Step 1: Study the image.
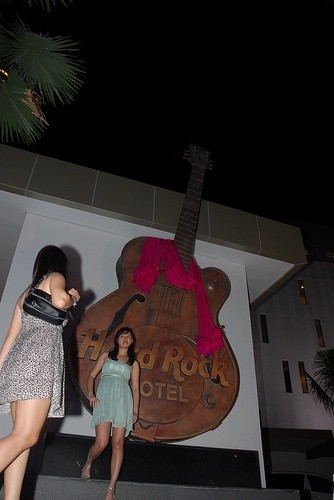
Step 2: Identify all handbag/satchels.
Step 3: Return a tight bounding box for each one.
[23,274,66,325]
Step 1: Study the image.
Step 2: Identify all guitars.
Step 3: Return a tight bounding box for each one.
[63,143,240,442]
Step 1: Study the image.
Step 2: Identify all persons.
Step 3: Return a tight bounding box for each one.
[0,244,81,500]
[81,327,140,499]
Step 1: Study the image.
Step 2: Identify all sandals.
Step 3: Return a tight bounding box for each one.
[81,465,91,480]
[104,488,115,500]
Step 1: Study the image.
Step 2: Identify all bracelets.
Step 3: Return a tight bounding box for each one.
[71,295,77,307]
